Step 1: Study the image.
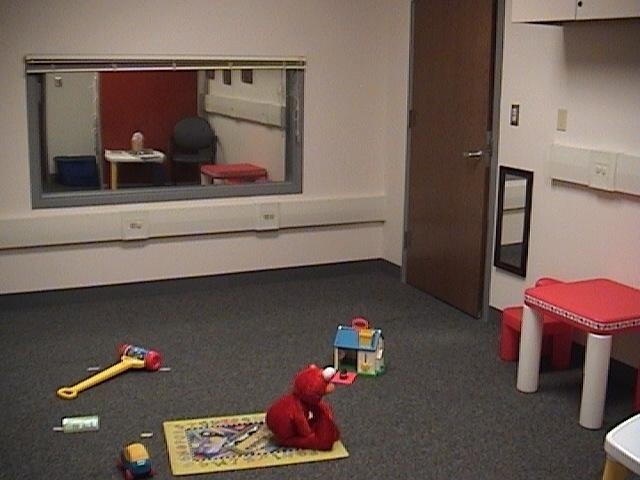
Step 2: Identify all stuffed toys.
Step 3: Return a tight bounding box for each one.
[265,361,341,453]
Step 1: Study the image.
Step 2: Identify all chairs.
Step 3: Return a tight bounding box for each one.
[501,279,572,369]
[166,115,217,186]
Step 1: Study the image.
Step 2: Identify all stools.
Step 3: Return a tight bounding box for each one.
[602,414,640,480]
[105,149,166,190]
[515,278,640,429]
[200,164,268,185]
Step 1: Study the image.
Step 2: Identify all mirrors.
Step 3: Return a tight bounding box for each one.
[493,165,534,278]
[23,54,305,209]
